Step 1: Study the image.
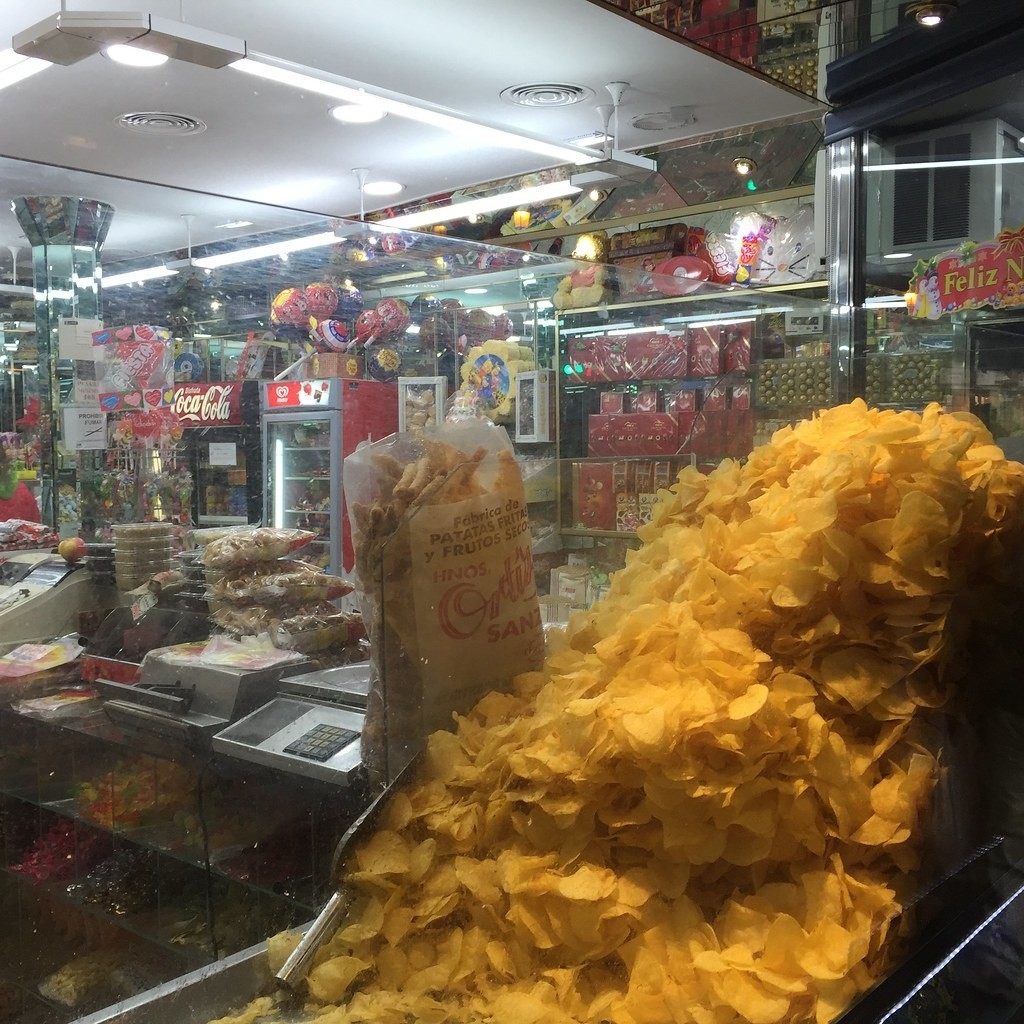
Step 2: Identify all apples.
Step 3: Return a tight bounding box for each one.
[59,537,84,562]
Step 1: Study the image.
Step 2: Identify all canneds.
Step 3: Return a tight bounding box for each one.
[200,448,246,516]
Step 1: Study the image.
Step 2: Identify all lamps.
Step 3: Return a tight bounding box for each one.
[0,0,656,300]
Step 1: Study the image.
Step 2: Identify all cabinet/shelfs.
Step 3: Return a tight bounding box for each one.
[555,280,955,574]
[0,690,374,1024]
[522,457,562,596]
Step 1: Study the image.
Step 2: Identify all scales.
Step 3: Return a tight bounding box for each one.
[211,659,372,787]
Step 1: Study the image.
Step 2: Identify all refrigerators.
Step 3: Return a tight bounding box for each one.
[172,379,262,530]
[259,377,398,612]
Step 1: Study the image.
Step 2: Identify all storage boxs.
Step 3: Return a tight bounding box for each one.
[569,322,752,384]
[589,383,755,457]
[572,462,717,531]
[398,376,448,432]
[515,369,555,443]
[303,353,363,379]
[680,7,762,71]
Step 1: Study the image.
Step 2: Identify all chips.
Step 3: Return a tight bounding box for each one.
[202,398,1024,1024]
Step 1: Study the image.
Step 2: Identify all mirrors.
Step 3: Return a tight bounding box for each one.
[605,0,910,109]
[171,299,555,396]
